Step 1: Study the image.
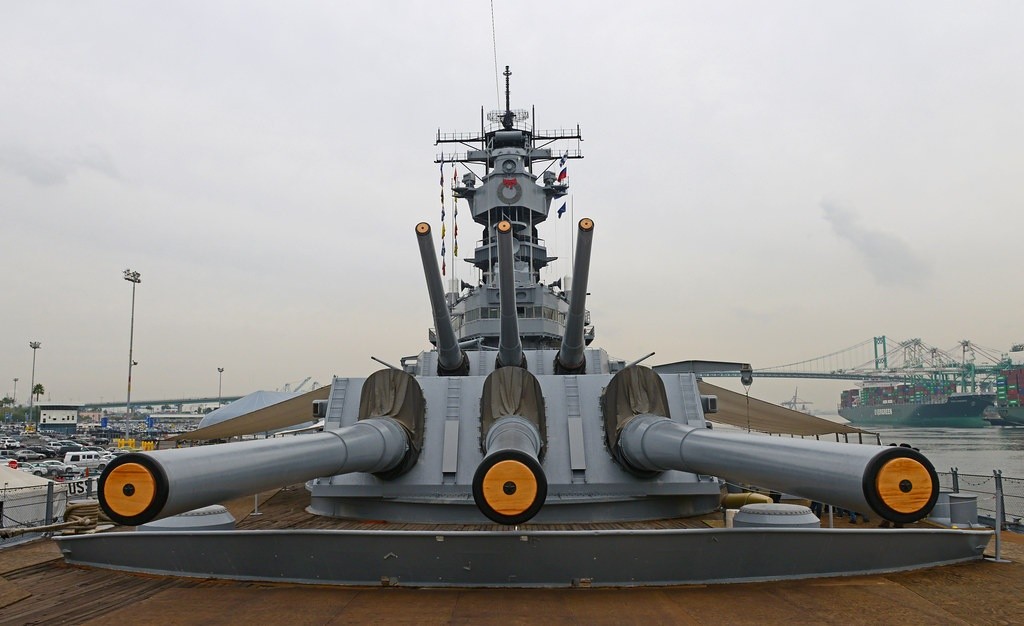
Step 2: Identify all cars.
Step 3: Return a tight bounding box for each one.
[45,460,78,476]
[0,420,111,452]
[15,450,47,460]
[39,461,65,475]
[17,462,48,477]
[100,452,126,462]
[0,450,29,462]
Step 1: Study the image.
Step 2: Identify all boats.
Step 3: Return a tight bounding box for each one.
[777,387,816,416]
[990,359,1024,428]
[836,387,995,428]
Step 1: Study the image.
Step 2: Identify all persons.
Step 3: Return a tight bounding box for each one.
[810,443,920,525]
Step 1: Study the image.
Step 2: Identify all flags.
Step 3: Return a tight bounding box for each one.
[557,203,566,219]
[559,150,568,168]
[558,168,566,182]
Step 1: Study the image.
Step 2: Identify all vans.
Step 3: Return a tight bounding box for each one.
[64,451,108,471]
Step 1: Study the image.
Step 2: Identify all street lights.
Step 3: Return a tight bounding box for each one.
[12,378,19,400]
[217,367,225,407]
[122,267,141,437]
[28,342,42,420]
